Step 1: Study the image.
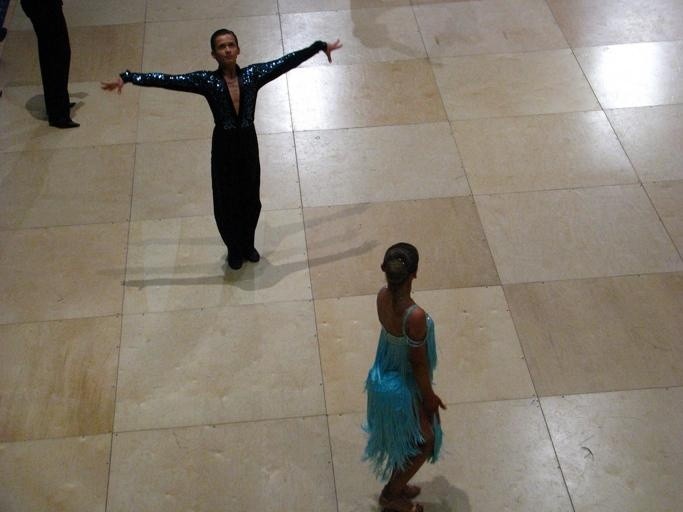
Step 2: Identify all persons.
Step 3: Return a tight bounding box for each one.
[2,0,84,130]
[360,242,448,512]
[99,28,344,271]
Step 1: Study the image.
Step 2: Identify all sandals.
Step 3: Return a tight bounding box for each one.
[379,486,423,512]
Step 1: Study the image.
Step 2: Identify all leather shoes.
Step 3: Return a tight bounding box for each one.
[227,246,260,270]
[47,102,81,128]
